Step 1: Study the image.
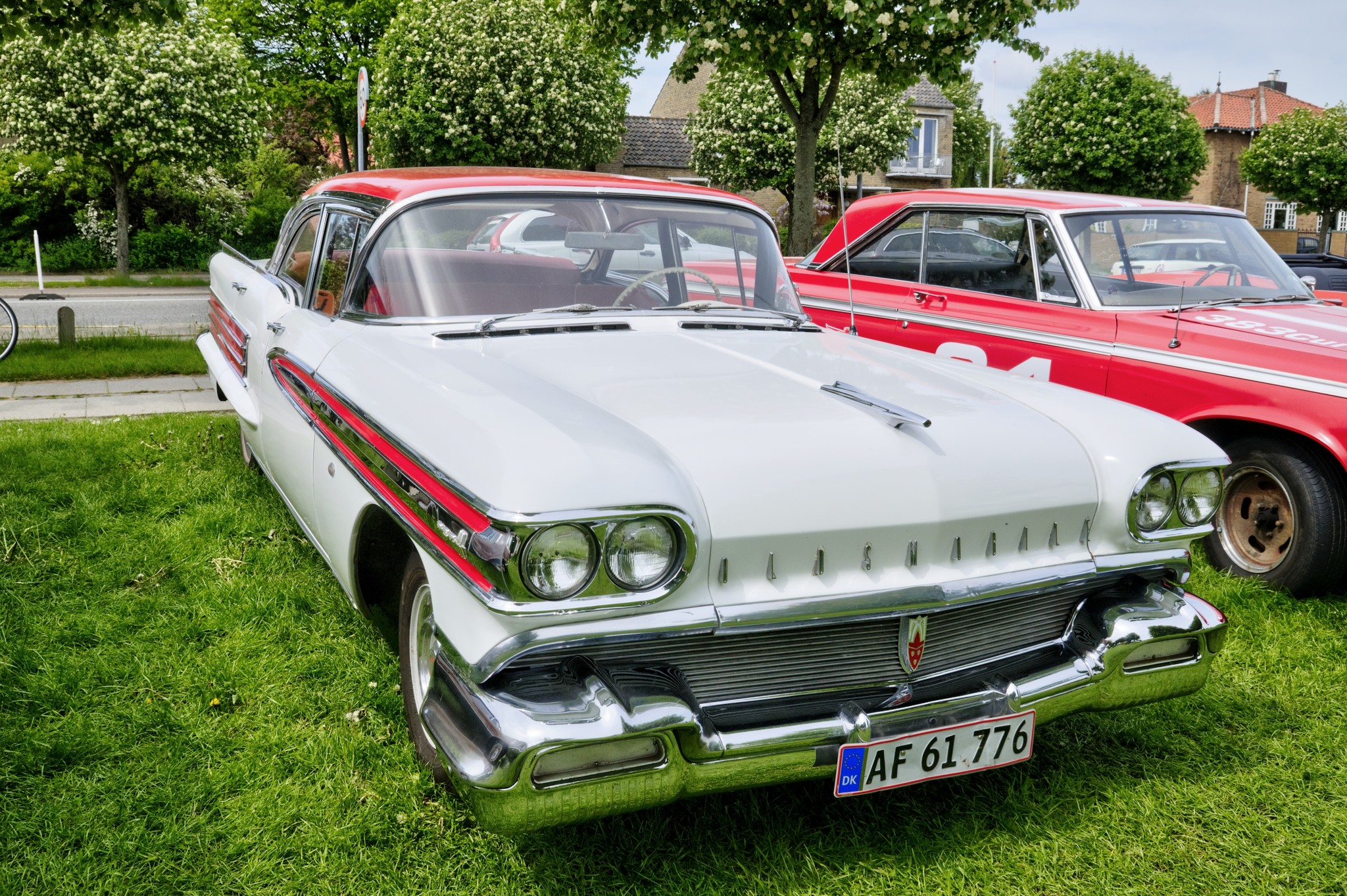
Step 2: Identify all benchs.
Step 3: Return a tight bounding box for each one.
[364,284,669,316]
[850,257,1033,302]
[286,246,578,284]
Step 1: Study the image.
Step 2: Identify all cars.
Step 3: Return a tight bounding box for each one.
[191,162,1232,840]
[658,185,1347,600]
[853,225,1018,260]
[1110,238,1281,288]
[466,209,757,270]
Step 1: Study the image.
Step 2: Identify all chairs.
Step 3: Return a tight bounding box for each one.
[899,159,911,174]
[929,158,945,174]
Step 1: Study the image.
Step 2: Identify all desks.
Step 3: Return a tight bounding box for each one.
[910,156,931,174]
